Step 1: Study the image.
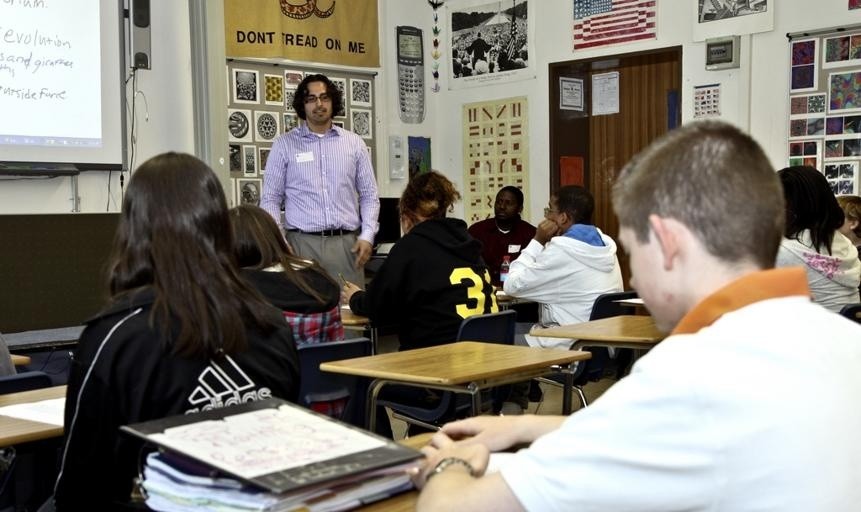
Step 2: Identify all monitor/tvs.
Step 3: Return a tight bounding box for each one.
[373,197,401,256]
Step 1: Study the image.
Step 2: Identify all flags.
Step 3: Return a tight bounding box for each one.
[573,0,655,50]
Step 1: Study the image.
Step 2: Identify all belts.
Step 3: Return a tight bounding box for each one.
[289,229,351,236]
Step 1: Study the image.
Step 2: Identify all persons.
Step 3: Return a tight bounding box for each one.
[0,332,18,379]
[451,23,527,79]
[774,164,861,325]
[341,171,623,442]
[259,74,381,292]
[411,121,861,510]
[31,121,351,510]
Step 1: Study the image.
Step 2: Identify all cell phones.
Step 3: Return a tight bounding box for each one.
[396,25,427,124]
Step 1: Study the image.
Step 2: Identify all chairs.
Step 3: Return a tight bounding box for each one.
[291,334,375,428]
[839,302,861,324]
[525,286,643,416]
[375,309,521,442]
[1,367,55,504]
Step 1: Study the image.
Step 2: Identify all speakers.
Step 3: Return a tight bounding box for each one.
[128,0,151,70]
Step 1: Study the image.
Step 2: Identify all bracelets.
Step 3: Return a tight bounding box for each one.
[425,457,473,481]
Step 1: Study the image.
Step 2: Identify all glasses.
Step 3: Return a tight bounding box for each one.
[544,207,571,221]
[304,94,330,103]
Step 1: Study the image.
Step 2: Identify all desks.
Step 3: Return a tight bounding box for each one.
[530,313,666,416]
[330,424,472,512]
[319,336,592,433]
[0,383,67,450]
[336,305,370,338]
[489,284,538,313]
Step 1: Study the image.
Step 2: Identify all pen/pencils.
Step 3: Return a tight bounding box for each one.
[339,272,349,288]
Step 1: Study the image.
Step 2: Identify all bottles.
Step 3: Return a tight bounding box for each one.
[499,254,511,289]
